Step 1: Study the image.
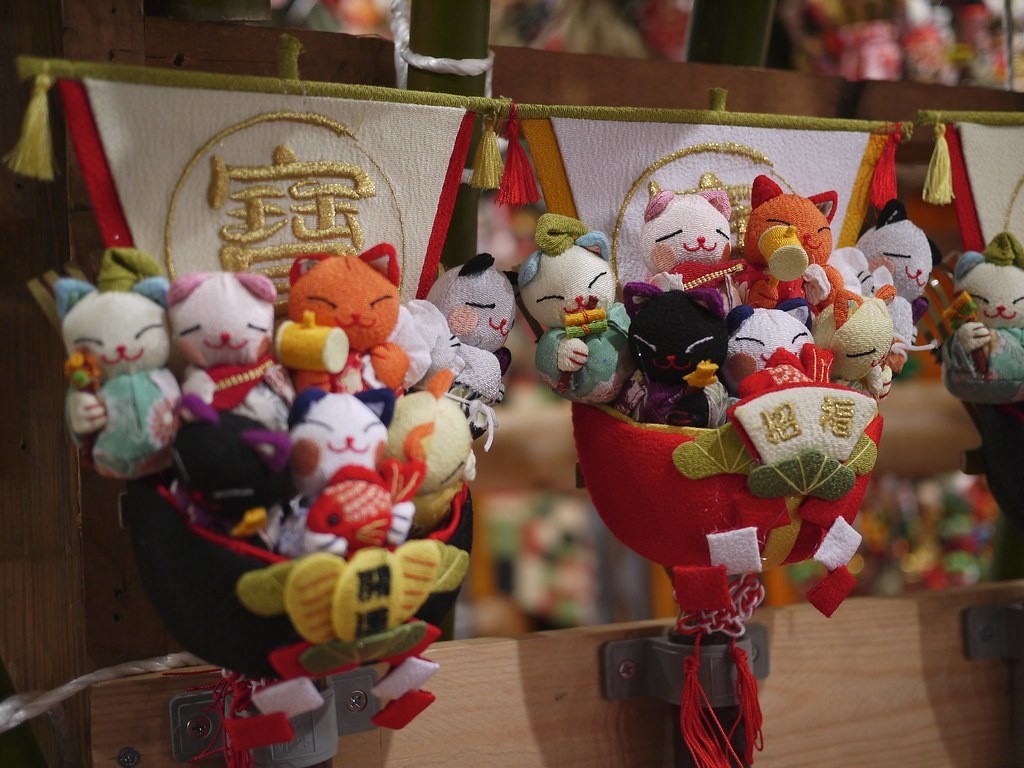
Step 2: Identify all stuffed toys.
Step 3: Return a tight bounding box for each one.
[518,175,943,430]
[55,243,519,564]
[940,231,1024,404]
